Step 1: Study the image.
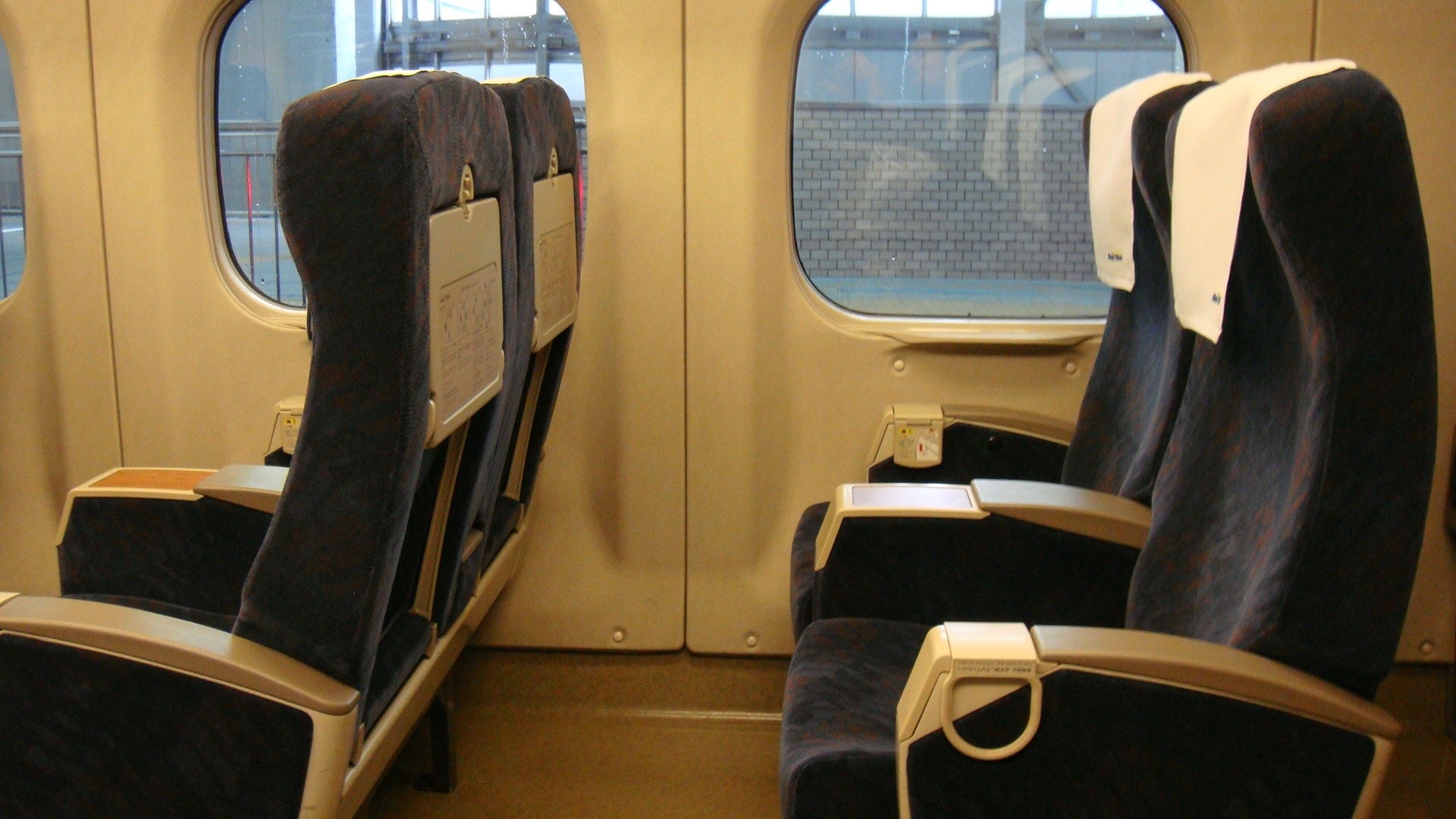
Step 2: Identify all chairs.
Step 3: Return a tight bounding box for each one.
[778,61,1435,819]
[0,69,589,819]
